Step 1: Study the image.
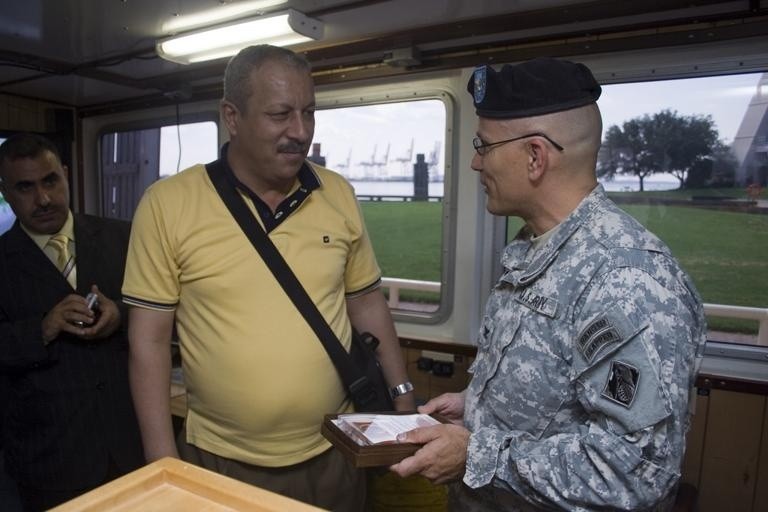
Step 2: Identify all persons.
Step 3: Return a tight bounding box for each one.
[0,135,143,511]
[388,60,706,511]
[121,43,417,512]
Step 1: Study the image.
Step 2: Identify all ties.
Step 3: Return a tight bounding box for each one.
[46,234,76,291]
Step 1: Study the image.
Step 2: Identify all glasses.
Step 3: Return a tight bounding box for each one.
[471,132,564,156]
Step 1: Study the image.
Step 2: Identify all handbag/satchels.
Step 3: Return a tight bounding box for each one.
[349,326,395,414]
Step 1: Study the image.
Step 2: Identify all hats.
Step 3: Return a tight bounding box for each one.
[466,56,602,118]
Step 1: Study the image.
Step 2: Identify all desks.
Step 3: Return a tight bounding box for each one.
[42,456,331,512]
[167,365,187,436]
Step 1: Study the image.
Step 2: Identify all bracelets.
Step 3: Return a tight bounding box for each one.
[388,382,415,400]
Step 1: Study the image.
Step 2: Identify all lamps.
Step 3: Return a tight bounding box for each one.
[153,8,325,66]
